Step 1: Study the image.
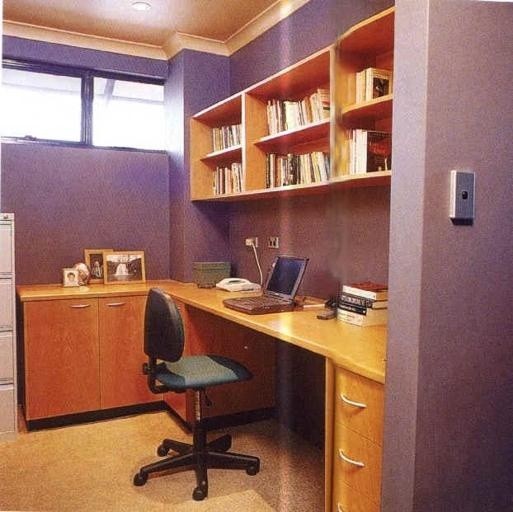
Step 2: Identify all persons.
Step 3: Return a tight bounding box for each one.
[90,260,102,279]
[64,271,77,285]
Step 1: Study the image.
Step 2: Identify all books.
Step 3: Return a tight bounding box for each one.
[209,67,391,195]
[335,279,388,327]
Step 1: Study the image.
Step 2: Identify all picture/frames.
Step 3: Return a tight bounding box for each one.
[82,247,146,286]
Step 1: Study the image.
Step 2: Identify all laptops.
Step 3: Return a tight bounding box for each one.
[223,254,309,315]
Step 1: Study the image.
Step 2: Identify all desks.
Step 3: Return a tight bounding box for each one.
[157,281,387,386]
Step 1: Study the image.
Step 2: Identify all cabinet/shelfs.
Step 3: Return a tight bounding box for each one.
[323,358,384,512]
[161,297,276,424]
[187,6,396,203]
[15,279,183,421]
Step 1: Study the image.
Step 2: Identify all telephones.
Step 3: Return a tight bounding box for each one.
[216,278,262,292]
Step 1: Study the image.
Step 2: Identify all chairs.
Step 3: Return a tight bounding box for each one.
[132,285,262,501]
